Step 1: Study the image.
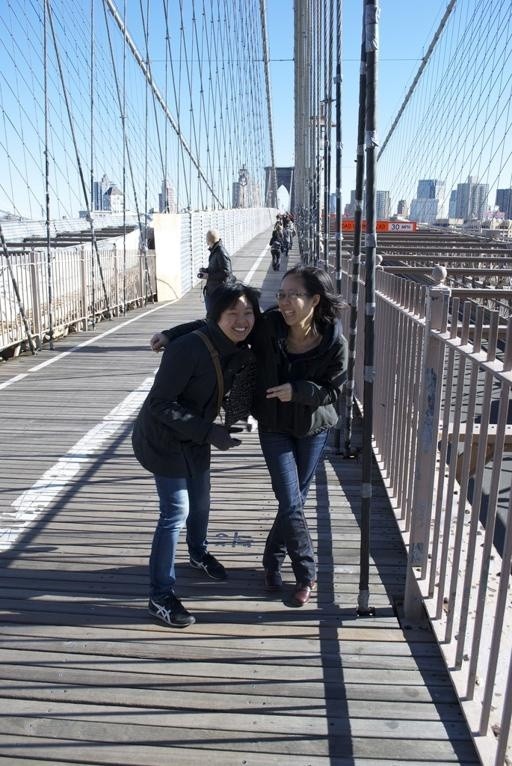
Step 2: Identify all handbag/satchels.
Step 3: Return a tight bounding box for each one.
[225,351,257,426]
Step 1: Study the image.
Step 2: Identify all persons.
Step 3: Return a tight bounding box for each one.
[197,229,233,314]
[269,211,297,272]
[150,266,350,606]
[130,276,261,627]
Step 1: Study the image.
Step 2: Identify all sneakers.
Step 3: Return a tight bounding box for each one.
[189,553,226,580]
[291,579,315,605]
[149,593,196,628]
[263,560,284,591]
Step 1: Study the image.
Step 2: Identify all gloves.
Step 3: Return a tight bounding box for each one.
[207,423,242,450]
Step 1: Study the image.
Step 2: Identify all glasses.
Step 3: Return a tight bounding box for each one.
[275,290,311,300]
